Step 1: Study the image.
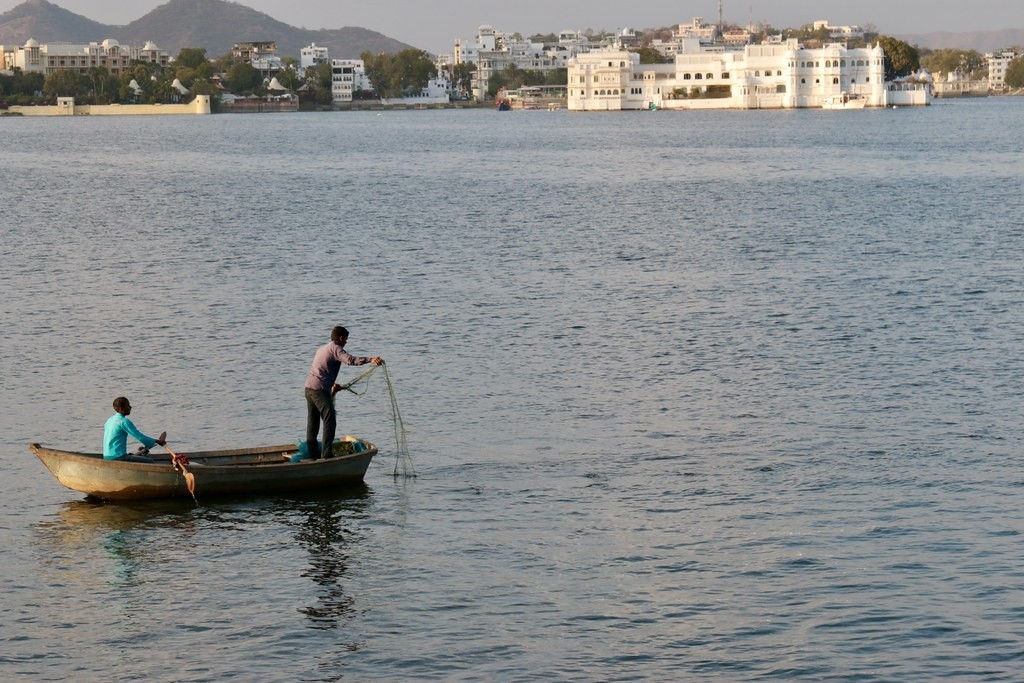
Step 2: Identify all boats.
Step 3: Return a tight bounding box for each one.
[28,436,380,505]
[820,92,869,110]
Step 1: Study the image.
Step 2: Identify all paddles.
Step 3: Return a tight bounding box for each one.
[159,431,195,494]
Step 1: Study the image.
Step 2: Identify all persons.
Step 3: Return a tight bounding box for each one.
[305,326,385,460]
[103,396,167,464]
